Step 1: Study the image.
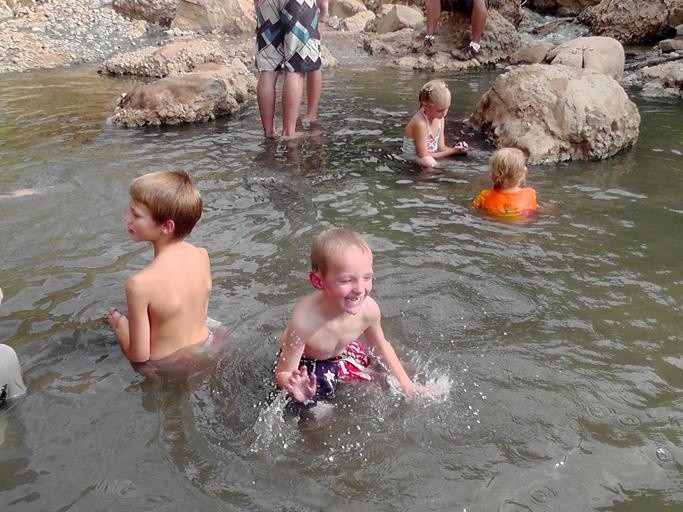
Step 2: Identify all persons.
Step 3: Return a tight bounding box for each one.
[256,0,322,159]
[272,228,438,436]
[0,288,27,447]
[297,0,327,144]
[402,80,469,171]
[104,168,228,383]
[423,0,492,60]
[470,147,540,228]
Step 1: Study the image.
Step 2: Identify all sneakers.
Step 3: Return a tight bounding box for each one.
[423,38,437,54]
[451,45,481,60]
